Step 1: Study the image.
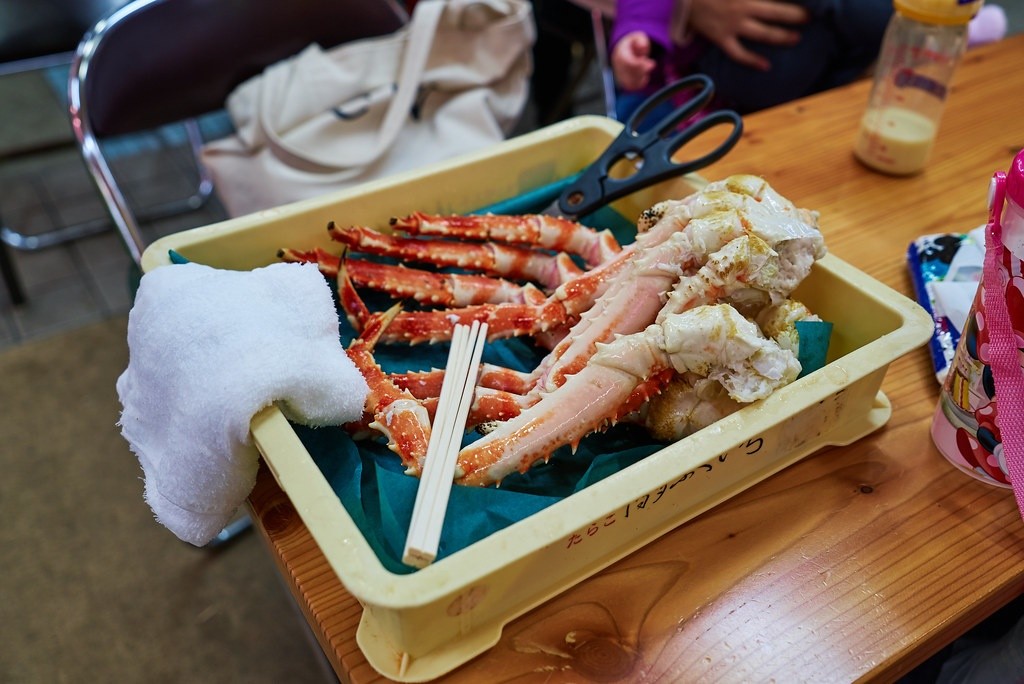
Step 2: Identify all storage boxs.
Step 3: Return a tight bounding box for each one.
[138,114,934,683]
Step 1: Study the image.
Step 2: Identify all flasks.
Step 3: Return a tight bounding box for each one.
[923,151,1024,496]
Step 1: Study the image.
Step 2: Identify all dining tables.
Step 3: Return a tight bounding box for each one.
[242,28,1024,682]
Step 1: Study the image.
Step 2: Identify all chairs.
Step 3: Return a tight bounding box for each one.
[65,0,410,261]
[1,1,211,305]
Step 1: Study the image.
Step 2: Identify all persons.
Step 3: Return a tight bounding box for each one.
[532,1,890,144]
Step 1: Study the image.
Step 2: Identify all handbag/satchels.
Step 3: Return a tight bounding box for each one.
[199,0,536,221]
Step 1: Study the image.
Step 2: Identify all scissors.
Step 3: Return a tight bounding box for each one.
[537,73,744,223]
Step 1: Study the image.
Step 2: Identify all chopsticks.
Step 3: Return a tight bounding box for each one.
[402,322,490,568]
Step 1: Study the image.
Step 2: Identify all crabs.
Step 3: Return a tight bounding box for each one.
[275,175,829,488]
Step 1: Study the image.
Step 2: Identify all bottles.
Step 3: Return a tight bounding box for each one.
[853,0,980,179]
